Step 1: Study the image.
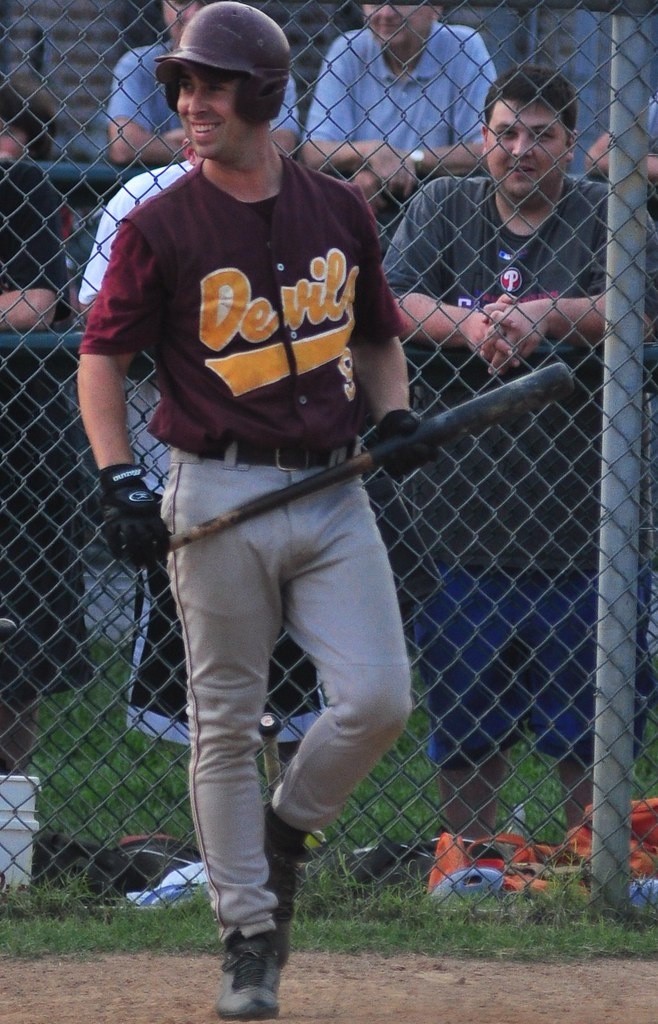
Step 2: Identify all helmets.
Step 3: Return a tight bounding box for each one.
[154,1,291,123]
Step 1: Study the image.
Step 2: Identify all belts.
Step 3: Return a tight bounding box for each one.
[198,434,357,471]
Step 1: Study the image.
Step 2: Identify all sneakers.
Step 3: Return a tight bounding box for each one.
[216,931,282,1019]
[263,800,308,970]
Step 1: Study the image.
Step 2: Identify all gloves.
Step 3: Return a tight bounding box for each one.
[98,463,172,574]
[378,409,442,466]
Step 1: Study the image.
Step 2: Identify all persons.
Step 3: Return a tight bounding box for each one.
[0,81,92,776]
[106,0,301,495]
[300,1,497,215]
[76,0,437,1024]
[380,65,658,842]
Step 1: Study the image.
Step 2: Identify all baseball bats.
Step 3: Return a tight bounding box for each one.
[165,357,579,554]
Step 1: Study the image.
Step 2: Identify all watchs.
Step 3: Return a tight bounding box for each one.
[409,150,425,171]
